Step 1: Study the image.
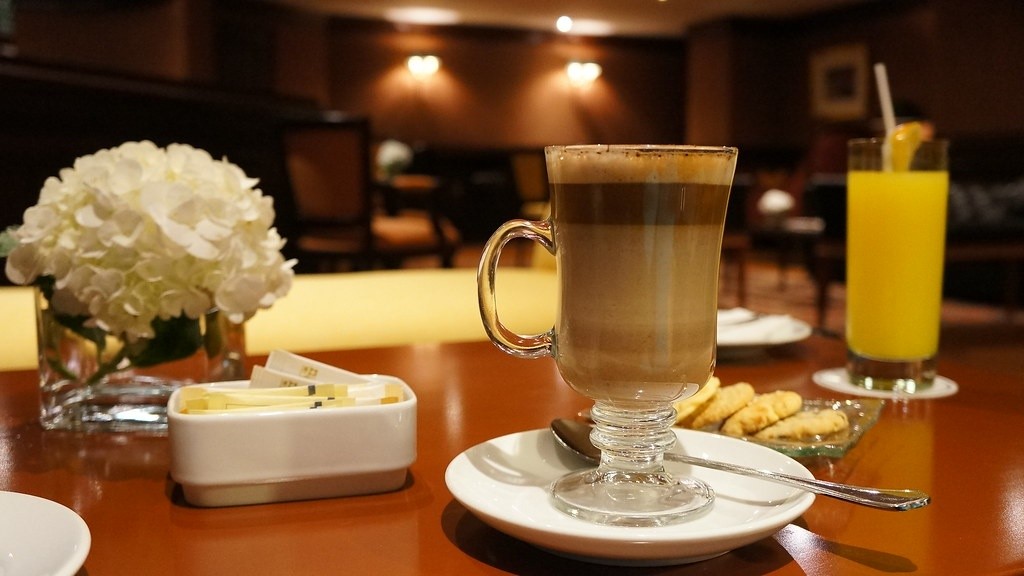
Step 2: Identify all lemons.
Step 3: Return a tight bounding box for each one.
[890,120,923,173]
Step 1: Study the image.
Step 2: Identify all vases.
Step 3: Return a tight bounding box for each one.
[34,276,244,436]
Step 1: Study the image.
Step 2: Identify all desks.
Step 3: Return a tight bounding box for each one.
[0,328,1024,576]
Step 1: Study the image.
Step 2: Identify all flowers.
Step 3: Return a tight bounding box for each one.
[5,141,295,334]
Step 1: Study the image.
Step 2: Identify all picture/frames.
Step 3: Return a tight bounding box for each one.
[809,39,875,122]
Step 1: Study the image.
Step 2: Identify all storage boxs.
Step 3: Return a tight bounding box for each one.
[167,373,419,508]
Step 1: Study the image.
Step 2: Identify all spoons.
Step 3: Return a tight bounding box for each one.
[551,420,932,512]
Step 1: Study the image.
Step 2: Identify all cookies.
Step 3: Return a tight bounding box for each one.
[668,377,850,438]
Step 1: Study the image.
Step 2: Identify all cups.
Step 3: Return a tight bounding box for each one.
[476,144,738,527]
[845,137,949,390]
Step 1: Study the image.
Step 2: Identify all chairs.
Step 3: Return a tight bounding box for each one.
[718,184,847,325]
[279,112,463,269]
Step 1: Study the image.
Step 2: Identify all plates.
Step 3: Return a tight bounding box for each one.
[714,398,886,460]
[717,308,813,359]
[0,492,92,576]
[445,426,817,565]
